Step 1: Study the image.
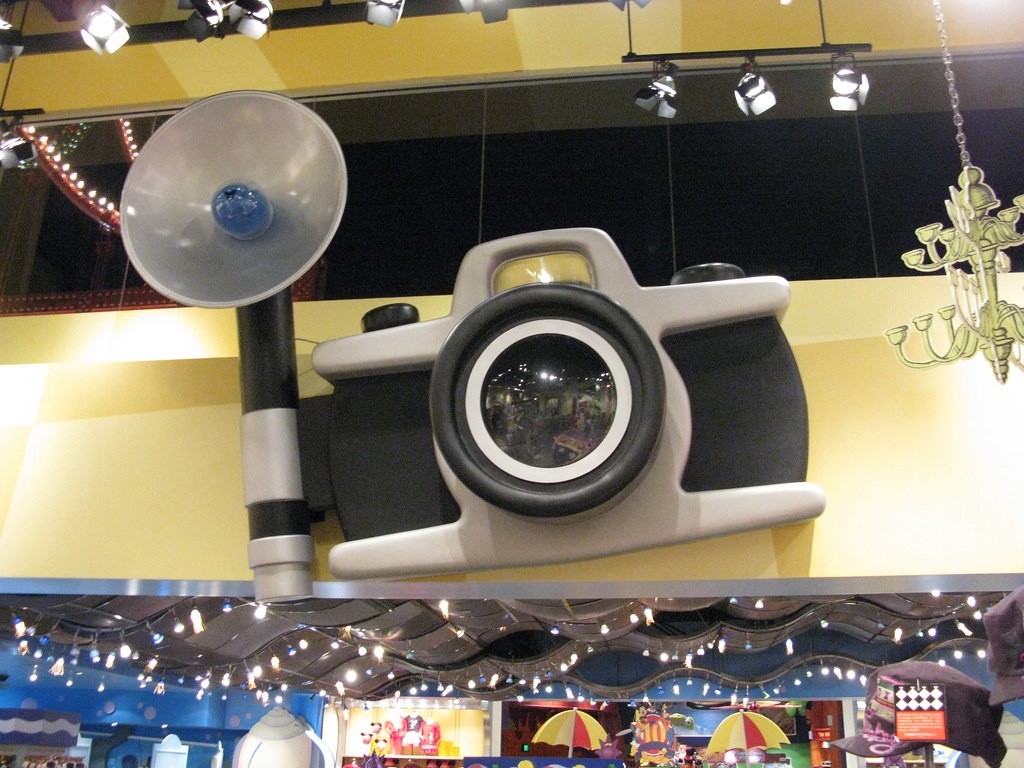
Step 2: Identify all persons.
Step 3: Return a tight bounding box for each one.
[361,711,442,754]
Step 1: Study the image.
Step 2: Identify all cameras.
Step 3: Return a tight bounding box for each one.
[309,226,833,580]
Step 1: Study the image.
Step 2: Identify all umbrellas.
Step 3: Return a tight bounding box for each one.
[706,709,791,768]
[531,707,610,759]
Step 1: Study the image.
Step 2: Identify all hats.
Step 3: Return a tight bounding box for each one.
[828,660,1007,768]
[983,586,1024,706]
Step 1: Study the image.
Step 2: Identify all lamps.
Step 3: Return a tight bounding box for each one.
[456,0,510,25]
[885,165,1024,384]
[178,0,276,44]
[633,63,680,120]
[733,56,776,118]
[359,0,406,28]
[609,0,652,11]
[826,50,871,113]
[44,0,135,54]
[0,11,27,67]
[0,114,43,172]
